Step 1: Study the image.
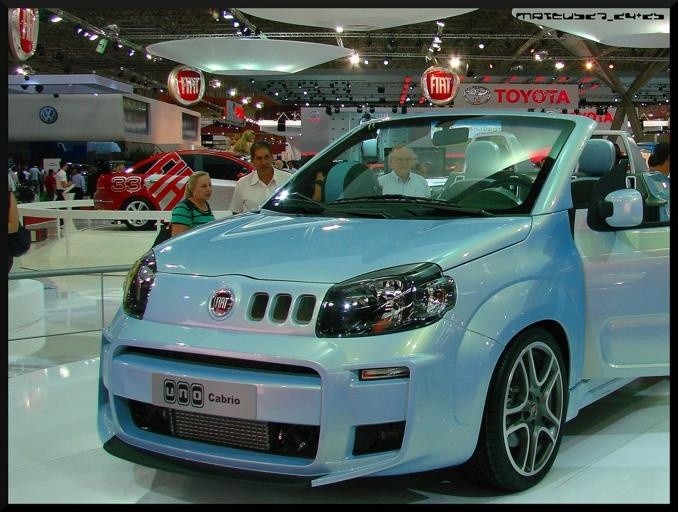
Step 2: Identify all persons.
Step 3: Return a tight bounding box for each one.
[377,145,431,199]
[229,129,257,156]
[647,154,661,171]
[650,142,671,179]
[8,162,124,212]
[287,161,298,175]
[171,171,216,237]
[229,141,291,214]
[274,159,284,170]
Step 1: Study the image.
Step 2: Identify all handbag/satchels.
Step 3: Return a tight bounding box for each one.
[151,201,193,249]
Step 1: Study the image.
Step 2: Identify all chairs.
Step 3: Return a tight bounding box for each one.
[572,138,631,209]
[465,142,504,181]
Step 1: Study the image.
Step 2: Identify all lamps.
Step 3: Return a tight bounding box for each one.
[44,8,566,110]
